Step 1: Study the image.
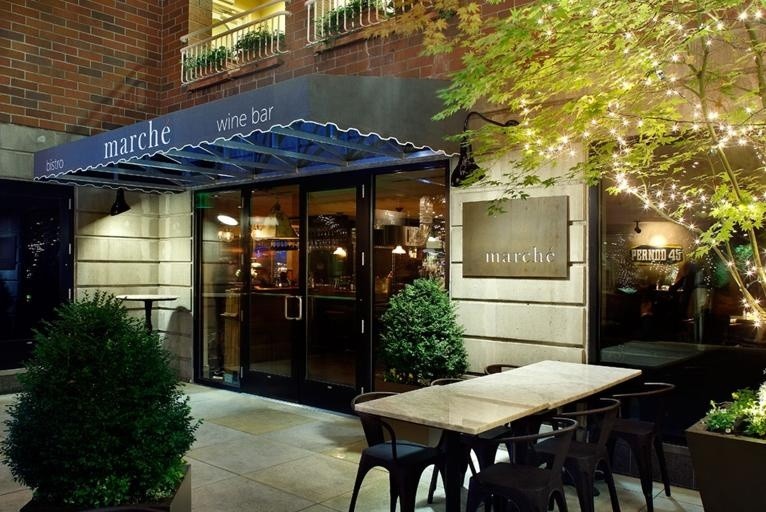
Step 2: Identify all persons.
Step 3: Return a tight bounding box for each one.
[281,268,296,286]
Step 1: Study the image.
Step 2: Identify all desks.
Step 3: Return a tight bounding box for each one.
[114,291,177,337]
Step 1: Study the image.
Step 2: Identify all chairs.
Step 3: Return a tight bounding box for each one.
[350,363,675,511]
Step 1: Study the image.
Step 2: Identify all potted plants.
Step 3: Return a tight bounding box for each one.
[312,1,426,38]
[684,379,765,512]
[373,272,470,446]
[181,29,286,82]
[0,287,204,512]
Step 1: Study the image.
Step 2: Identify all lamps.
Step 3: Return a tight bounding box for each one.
[451,110,521,189]
